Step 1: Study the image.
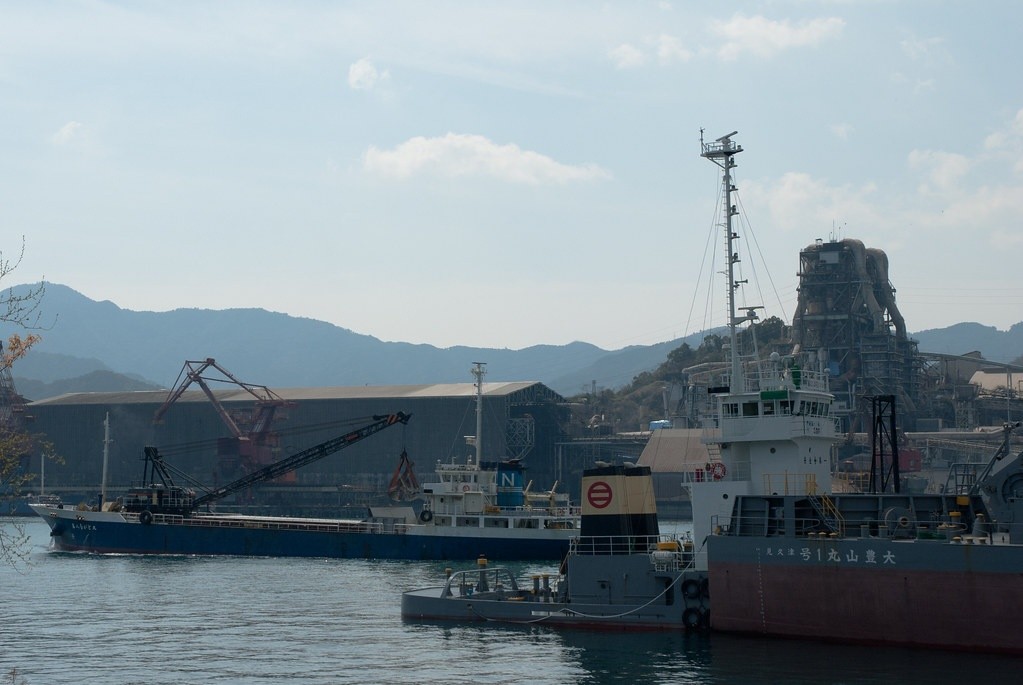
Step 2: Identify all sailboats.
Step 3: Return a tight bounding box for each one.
[27,361,585,561]
[682,131,844,569]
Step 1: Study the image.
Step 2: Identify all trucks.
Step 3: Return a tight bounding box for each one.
[649,420,672,432]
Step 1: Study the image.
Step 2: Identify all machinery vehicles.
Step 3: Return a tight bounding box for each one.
[120,412,413,516]
[153,358,287,465]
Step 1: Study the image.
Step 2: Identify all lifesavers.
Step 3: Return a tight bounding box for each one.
[139,510,153,526]
[681,579,711,631]
[711,462,727,479]
[463,485,470,492]
[420,509,433,522]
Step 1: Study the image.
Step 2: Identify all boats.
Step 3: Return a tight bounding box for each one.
[706,394,1021,654]
[396,465,709,641]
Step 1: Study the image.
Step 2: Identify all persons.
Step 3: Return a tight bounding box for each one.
[57,502,63,509]
